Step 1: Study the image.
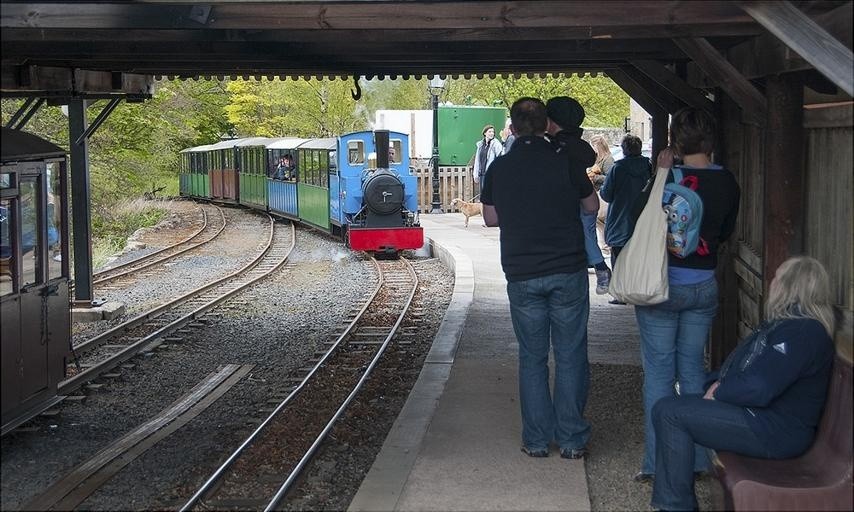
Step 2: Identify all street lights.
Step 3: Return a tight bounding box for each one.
[426,76,447,214]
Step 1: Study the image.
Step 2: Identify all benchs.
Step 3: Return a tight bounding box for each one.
[710,355,854,512]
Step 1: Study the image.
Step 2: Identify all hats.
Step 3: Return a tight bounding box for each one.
[545,96,585,129]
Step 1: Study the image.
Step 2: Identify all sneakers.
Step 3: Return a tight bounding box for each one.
[596,269,611,295]
[520,441,548,457]
[561,448,585,459]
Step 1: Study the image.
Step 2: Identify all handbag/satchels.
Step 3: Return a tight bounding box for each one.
[607,209,670,307]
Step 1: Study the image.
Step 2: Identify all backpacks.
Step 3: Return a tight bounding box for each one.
[666,169,710,259]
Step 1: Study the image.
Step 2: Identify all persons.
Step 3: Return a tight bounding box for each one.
[650,254,834,511]
[586,134,616,273]
[473,125,503,183]
[600,133,652,306]
[502,118,516,153]
[545,96,612,295]
[278,154,297,180]
[388,147,396,163]
[626,107,741,485]
[479,96,599,459]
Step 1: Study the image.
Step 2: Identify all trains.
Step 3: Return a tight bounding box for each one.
[177,127,424,259]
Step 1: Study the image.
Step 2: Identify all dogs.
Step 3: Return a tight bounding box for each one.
[449,197,483,229]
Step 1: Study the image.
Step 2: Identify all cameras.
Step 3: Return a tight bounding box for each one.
[673,153,683,164]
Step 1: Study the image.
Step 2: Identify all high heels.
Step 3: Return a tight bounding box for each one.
[636,474,655,487]
